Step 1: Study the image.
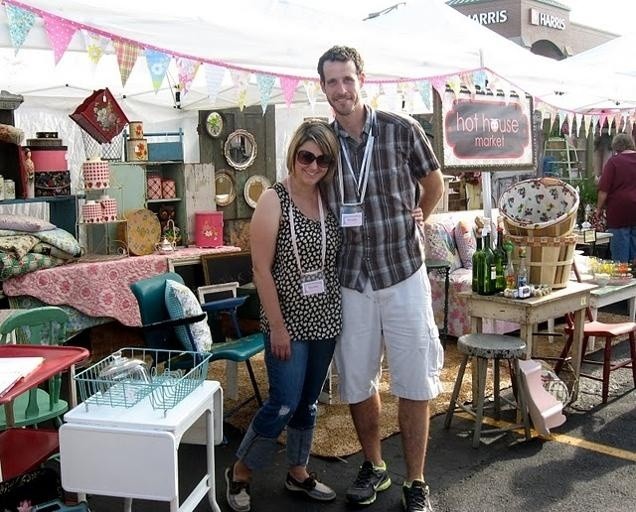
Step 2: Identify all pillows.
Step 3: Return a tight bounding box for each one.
[424,214,463,273]
[0,217,57,232]
[453,217,476,269]
[164,276,214,353]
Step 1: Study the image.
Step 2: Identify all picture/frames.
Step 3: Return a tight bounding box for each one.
[200,249,261,340]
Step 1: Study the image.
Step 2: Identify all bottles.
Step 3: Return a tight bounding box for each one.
[0,175,15,201]
[517,246,529,288]
[505,251,515,288]
[471,229,507,296]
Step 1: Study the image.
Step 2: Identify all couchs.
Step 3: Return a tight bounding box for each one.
[420,208,520,340]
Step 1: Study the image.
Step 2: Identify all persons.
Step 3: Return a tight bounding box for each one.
[591,131,636,268]
[224,118,426,510]
[312,48,447,511]
[22,147,39,201]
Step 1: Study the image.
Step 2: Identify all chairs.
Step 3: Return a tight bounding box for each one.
[551,258,636,403]
[133,272,272,427]
[0,306,69,435]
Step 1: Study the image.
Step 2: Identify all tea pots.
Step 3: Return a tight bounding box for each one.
[153,236,176,254]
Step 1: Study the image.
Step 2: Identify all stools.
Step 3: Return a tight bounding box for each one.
[442,332,533,449]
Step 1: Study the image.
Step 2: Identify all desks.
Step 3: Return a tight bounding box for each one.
[574,272,636,350]
[463,283,596,443]
[3,243,242,342]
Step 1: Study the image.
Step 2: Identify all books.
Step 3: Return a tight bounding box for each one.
[0,355,45,399]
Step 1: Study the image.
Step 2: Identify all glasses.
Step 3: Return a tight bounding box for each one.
[296,150,331,168]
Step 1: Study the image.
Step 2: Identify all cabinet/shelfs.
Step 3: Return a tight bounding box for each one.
[0,342,85,512]
[71,161,129,262]
[0,89,50,221]
[424,256,451,347]
[58,377,226,512]
[107,162,188,248]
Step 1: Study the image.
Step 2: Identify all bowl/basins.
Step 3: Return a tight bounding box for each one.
[596,274,610,288]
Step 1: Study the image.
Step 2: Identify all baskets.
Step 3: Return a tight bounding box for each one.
[71,347,212,417]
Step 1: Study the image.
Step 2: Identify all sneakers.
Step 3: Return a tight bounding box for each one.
[401,478,433,512]
[225,467,252,511]
[346,459,392,507]
[285,472,337,501]
